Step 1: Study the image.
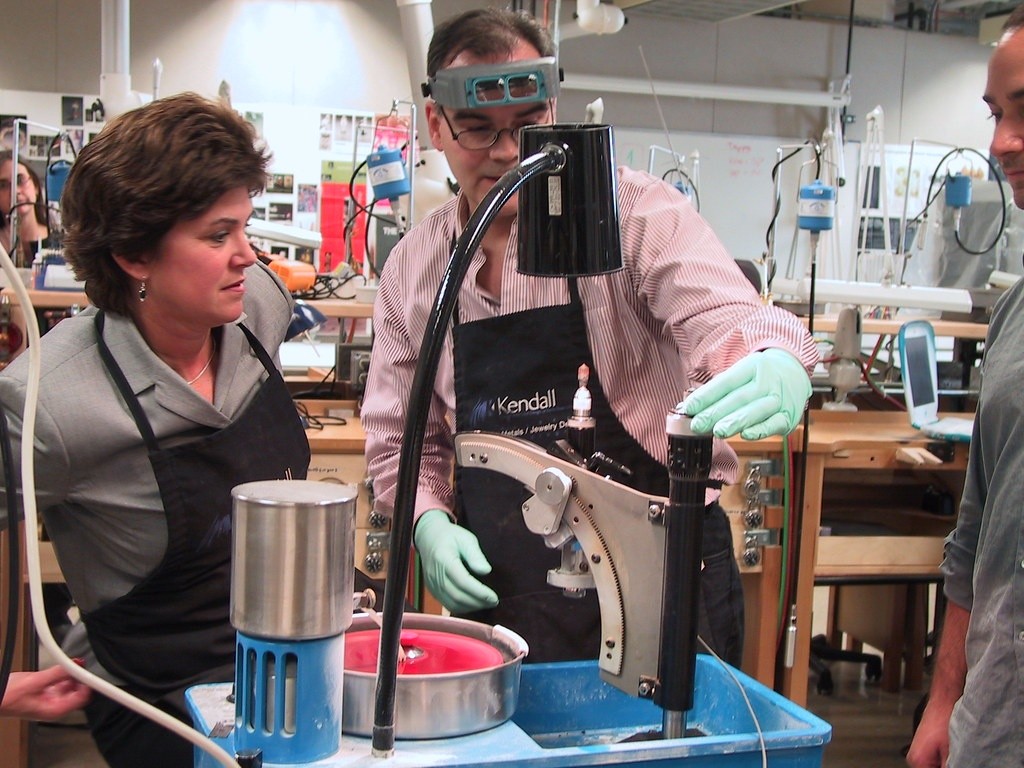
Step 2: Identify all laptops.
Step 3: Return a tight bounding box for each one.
[898,320,975,441]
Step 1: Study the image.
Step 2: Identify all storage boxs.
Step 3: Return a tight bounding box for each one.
[184,654,832,768]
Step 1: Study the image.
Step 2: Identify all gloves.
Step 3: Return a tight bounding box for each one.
[416,509,499,614]
[678,348,813,440]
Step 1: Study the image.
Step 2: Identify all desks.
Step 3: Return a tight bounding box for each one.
[0,287,989,768]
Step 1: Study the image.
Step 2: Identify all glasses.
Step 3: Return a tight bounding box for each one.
[440,101,555,150]
[0,174,33,190]
[465,69,549,107]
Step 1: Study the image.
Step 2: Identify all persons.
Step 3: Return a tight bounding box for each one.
[907,2,1024,768]
[0,153,66,269]
[359,7,818,665]
[0,92,311,768]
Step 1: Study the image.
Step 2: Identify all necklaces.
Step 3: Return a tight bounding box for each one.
[187,337,217,385]
